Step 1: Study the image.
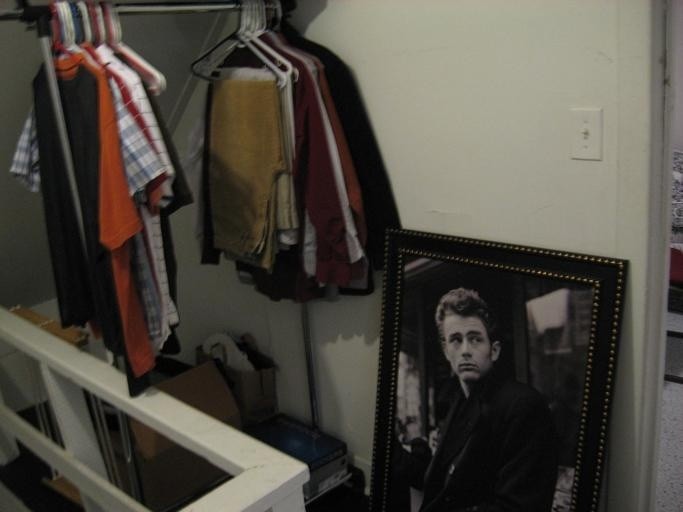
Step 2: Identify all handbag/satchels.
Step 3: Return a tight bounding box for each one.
[194,329,280,428]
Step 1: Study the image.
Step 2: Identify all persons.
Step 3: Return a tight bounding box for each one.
[396,418,429,511]
[425,427,440,456]
[397,288,559,511]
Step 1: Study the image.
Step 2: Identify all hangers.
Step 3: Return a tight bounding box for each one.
[52,1,301,98]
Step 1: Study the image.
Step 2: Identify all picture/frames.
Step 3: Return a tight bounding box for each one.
[366,224,630,512]
